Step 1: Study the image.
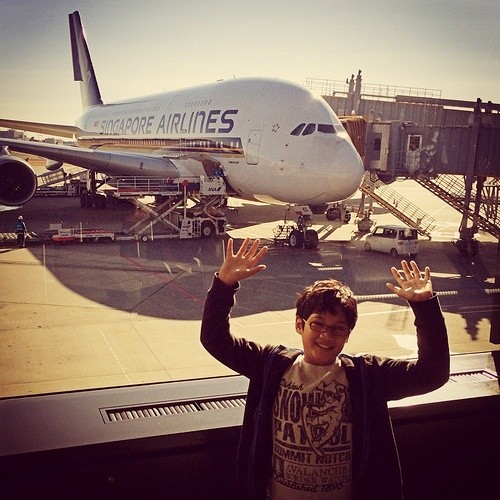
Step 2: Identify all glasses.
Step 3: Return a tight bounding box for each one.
[302,319,351,336]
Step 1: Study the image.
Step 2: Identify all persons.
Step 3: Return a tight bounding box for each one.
[345,73,355,99]
[200,236,450,500]
[355,69,363,101]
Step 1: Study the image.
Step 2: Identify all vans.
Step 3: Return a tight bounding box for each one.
[364,224,419,259]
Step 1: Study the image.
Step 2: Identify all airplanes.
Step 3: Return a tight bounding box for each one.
[0,11,363,249]
